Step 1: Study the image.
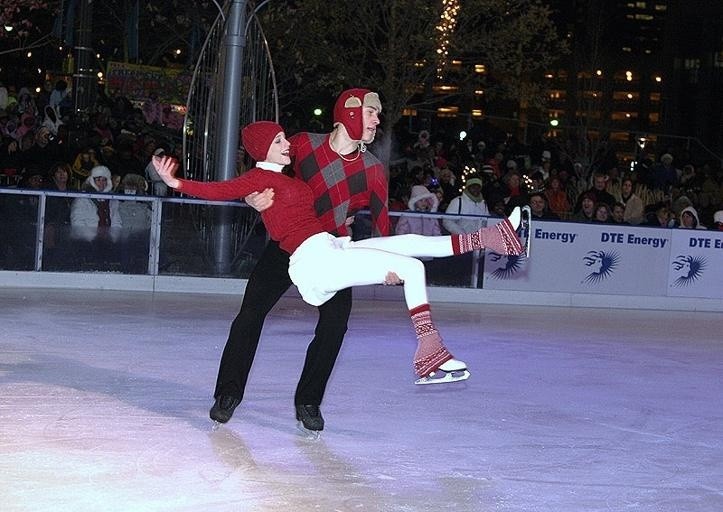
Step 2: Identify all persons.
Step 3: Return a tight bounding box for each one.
[235,144,246,178]
[2,77,184,275]
[388,127,723,231]
[149,120,533,386]
[394,176,490,287]
[206,86,403,433]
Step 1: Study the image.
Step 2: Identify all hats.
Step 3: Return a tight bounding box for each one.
[581,193,596,206]
[333,89,382,144]
[466,176,482,190]
[241,121,284,161]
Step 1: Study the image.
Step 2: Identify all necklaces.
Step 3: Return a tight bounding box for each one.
[330,132,361,162]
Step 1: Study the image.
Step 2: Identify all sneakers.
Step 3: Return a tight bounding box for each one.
[429,358,467,377]
[296,404,324,430]
[210,395,241,423]
[508,206,521,232]
